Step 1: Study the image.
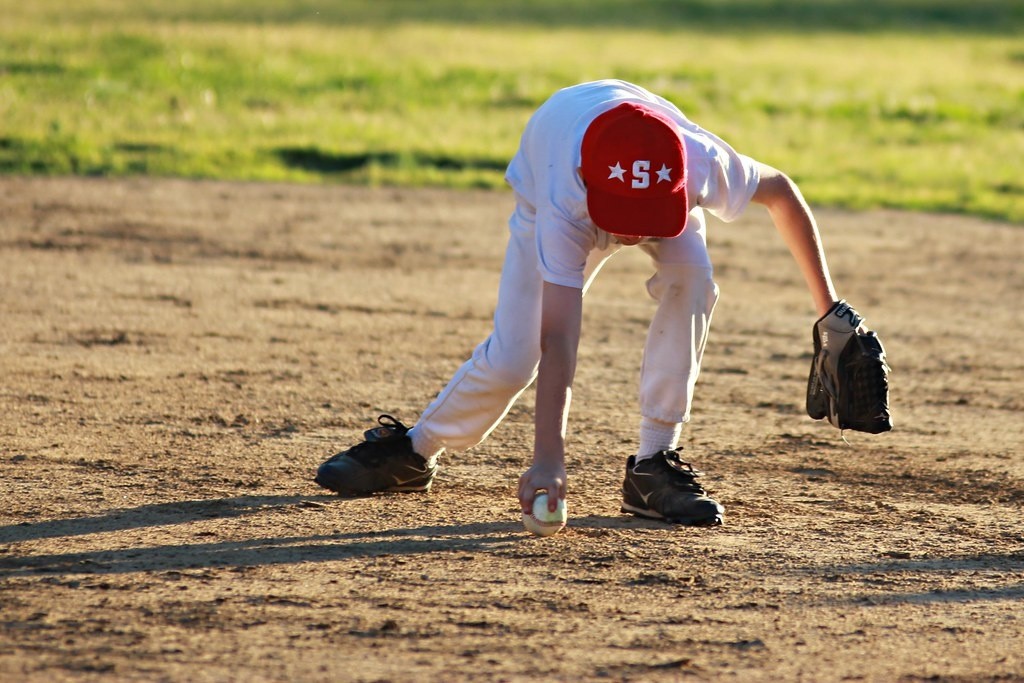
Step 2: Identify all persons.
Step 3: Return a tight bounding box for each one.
[314,79,892,528]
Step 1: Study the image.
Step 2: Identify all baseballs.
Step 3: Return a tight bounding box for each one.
[521,491,568,538]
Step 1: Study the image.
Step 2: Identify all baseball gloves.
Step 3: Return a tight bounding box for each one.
[803,299,895,436]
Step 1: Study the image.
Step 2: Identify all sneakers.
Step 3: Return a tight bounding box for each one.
[314,414,439,492]
[620,446,725,526]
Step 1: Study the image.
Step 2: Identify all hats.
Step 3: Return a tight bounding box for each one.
[579,103,689,239]
